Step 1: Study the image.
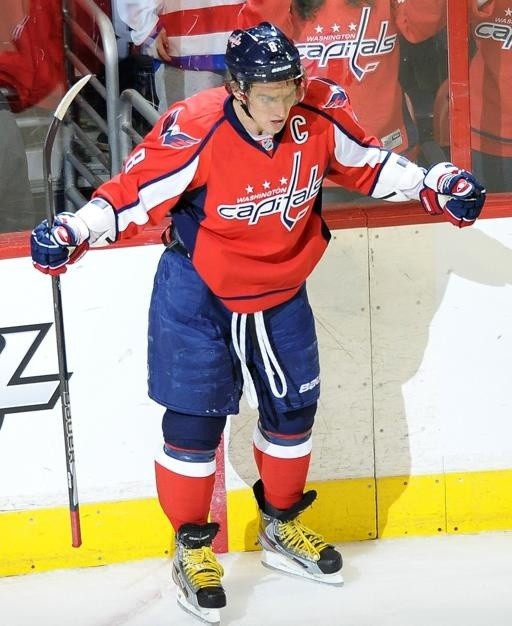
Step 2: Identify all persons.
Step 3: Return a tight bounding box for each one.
[28,19,488,612]
[0,0,511,201]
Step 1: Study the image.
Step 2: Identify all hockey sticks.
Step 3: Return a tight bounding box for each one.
[44,74,94,549]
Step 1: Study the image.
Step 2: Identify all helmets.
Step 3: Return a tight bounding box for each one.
[226,22,308,105]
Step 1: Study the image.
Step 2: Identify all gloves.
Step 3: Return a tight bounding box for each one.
[31,197,119,276]
[419,161,485,225]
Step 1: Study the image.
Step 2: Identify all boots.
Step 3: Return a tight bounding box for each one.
[253,479,342,573]
[174,521,226,610]
